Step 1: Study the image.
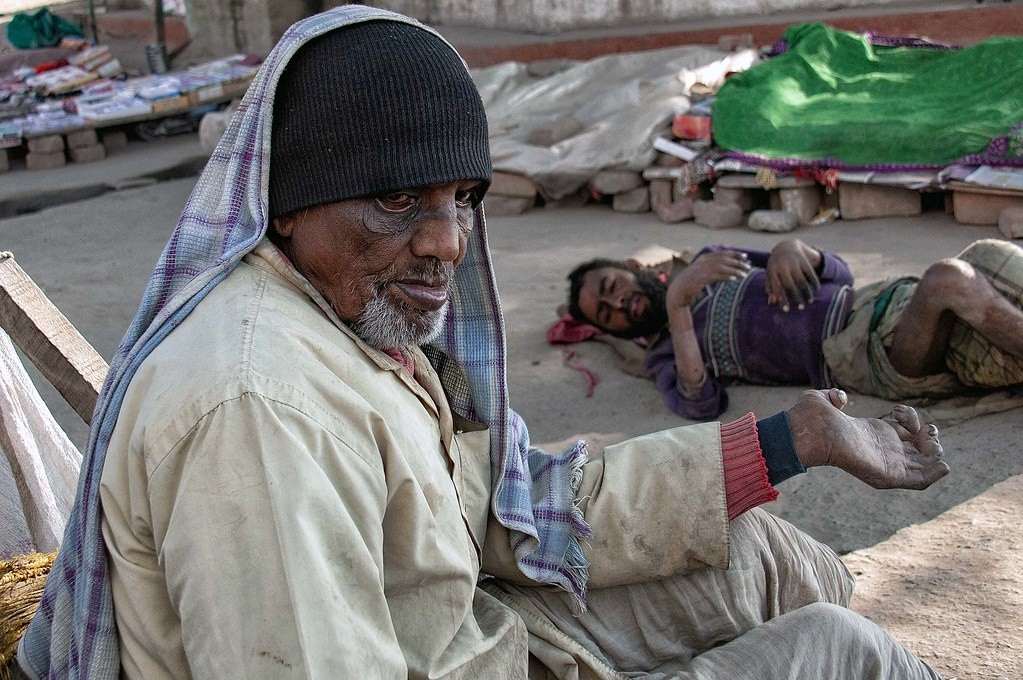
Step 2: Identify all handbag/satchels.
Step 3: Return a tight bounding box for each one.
[68,45,127,81]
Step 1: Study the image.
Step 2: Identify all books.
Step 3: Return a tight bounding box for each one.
[0,44,259,141]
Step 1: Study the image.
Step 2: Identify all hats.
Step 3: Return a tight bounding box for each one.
[267,18,492,211]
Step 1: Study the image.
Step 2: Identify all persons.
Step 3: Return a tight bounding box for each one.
[17,6,951,680]
[566,239,1023,421]
